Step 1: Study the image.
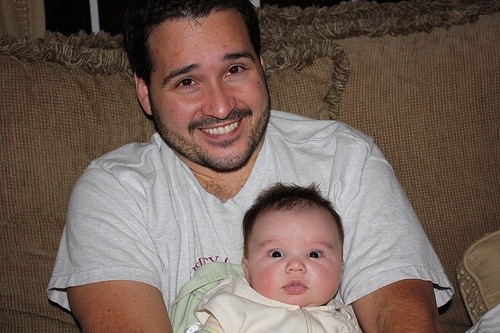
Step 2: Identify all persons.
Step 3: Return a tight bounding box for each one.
[194,181,365,333]
[48,0,455,332]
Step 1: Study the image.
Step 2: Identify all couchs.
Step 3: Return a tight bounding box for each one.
[0,0,500,332]
